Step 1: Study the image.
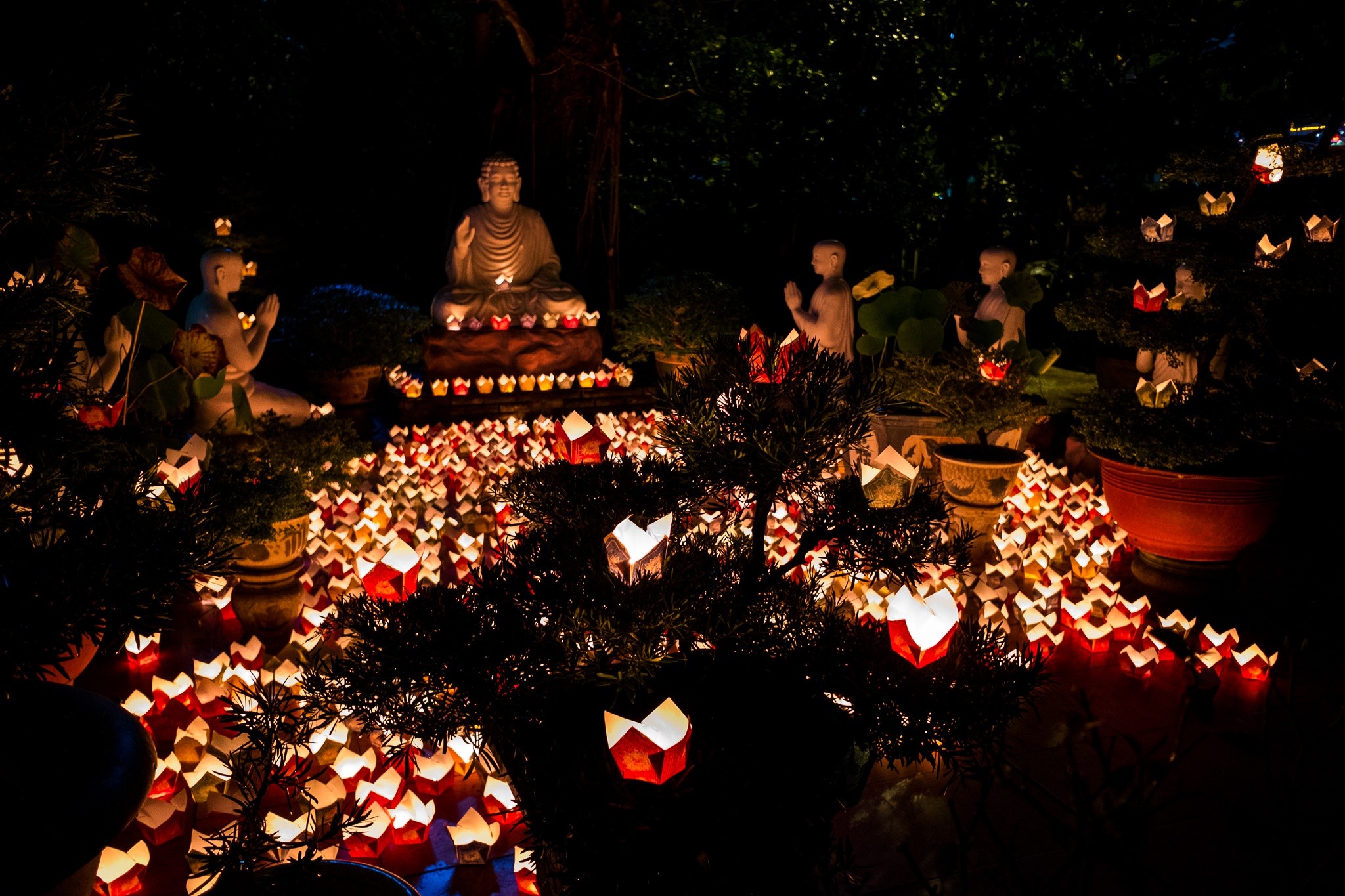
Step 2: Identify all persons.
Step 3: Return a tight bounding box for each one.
[953,246,1025,356]
[179,249,310,434]
[426,151,603,380]
[54,296,134,405]
[784,240,854,383]
[1136,263,1215,387]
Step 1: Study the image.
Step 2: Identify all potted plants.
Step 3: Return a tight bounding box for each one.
[0,80,1345,896]
